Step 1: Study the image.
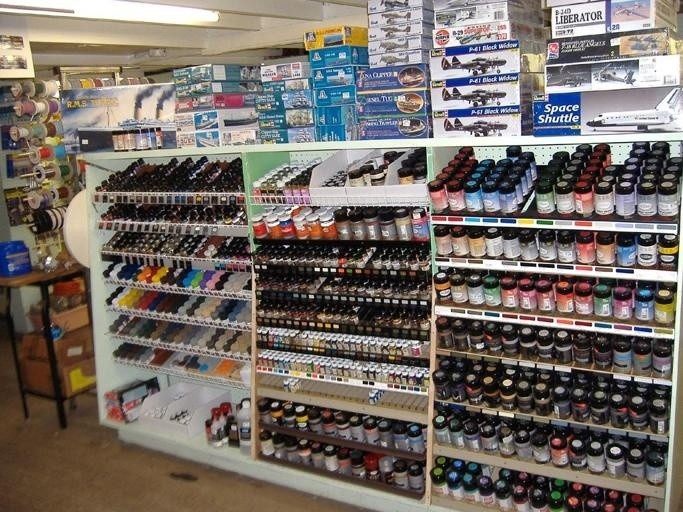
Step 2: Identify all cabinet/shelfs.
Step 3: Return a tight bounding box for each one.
[248,139,433,511]
[428,134,682,512]
[78,144,254,480]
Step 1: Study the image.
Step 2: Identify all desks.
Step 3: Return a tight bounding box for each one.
[0,265,92,429]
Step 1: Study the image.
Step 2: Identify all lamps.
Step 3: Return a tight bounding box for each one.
[72,1,220,24]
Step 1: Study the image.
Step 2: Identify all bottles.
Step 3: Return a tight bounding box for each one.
[204,397,251,456]
[251,141,683,511]
[111,126,164,153]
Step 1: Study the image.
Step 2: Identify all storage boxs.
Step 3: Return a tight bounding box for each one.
[21,325,93,366]
[20,357,95,399]
